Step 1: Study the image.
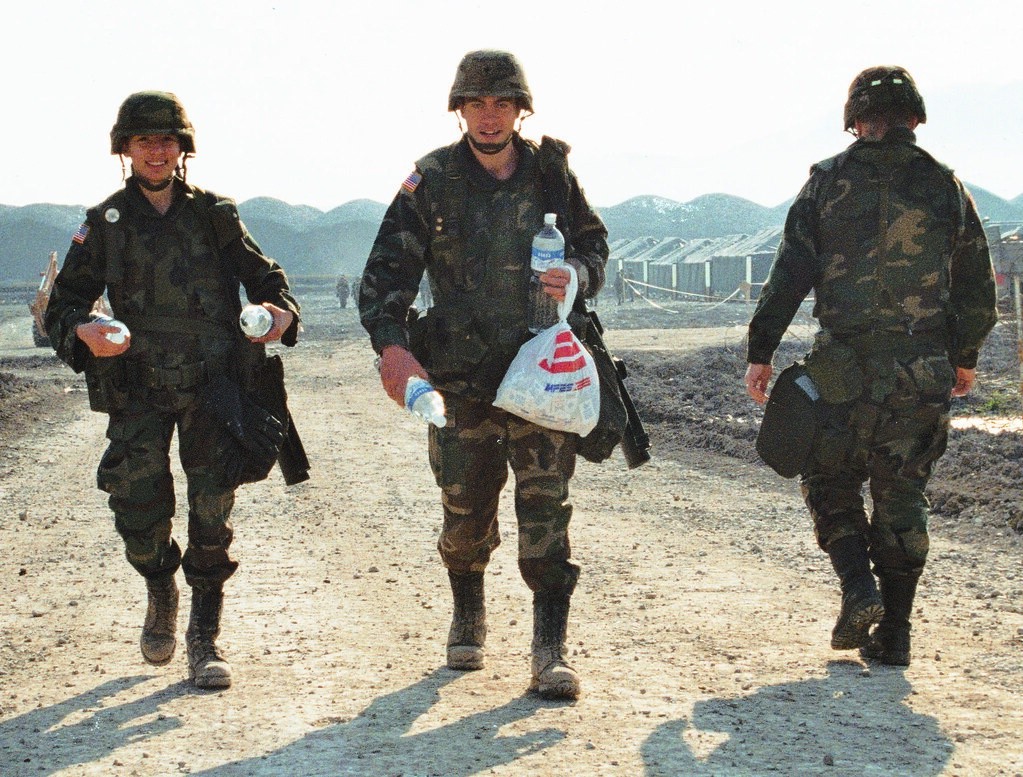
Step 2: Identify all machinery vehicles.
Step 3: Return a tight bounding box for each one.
[27,249,108,347]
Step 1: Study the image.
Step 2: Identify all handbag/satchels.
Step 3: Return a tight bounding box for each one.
[757,363,820,479]
[574,373,630,465]
[244,355,310,486]
[492,266,600,437]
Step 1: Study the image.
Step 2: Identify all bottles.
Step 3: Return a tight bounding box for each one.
[525,212,566,335]
[86,312,131,345]
[239,304,275,338]
[373,357,448,428]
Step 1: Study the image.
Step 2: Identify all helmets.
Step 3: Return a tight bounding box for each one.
[110,91,195,156]
[843,66,927,133]
[448,52,535,113]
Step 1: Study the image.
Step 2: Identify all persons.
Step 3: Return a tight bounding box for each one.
[45,89,299,688]
[356,49,610,702]
[336,272,361,310]
[744,65,1001,665]
[614,266,634,307]
[587,296,599,307]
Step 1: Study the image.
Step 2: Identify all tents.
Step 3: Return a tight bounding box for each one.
[606,228,782,302]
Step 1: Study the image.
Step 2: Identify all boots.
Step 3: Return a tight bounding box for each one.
[861,575,917,665]
[531,594,581,695]
[140,574,179,666]
[185,585,232,685]
[830,538,885,651]
[447,574,487,670]
